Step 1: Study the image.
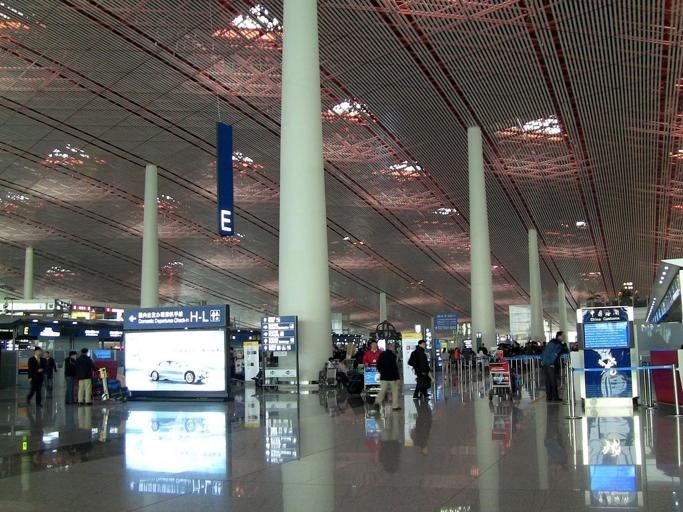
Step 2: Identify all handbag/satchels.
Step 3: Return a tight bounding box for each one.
[417,375,431,392]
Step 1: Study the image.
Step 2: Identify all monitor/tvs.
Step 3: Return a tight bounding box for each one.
[581,321,630,349]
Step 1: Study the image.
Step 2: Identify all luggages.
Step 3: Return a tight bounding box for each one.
[99,380,120,393]
[73,382,79,403]
[347,374,364,395]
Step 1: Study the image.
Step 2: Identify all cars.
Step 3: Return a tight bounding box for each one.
[144,412,214,437]
[148,359,210,385]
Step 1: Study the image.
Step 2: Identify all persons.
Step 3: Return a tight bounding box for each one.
[76,348,99,405]
[26,347,47,407]
[64,351,79,405]
[42,351,58,400]
[331,330,579,411]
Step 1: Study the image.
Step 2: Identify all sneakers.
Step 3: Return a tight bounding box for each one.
[375,404,379,411]
[78,403,93,407]
[413,396,420,398]
[392,408,401,410]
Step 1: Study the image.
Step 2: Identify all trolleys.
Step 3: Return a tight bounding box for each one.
[97,367,130,403]
[361,361,386,403]
[489,360,522,401]
